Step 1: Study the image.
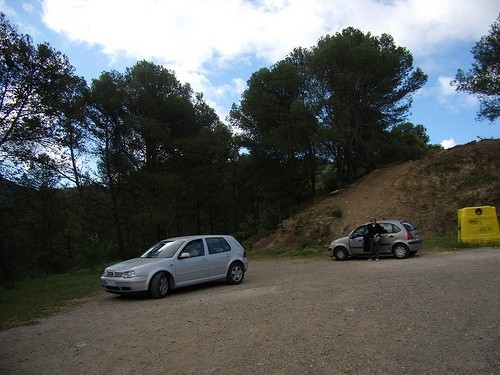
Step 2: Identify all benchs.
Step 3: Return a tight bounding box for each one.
[212,248,224,253]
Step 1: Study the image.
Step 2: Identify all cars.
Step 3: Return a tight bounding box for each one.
[99,235,249,300]
[326,219,424,261]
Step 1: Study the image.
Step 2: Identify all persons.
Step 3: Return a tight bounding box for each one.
[367,217,382,262]
[191,241,202,256]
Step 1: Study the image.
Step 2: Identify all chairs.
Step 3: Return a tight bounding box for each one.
[189,247,200,256]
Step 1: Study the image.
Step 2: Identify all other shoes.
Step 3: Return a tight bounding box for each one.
[375,258,380,262]
[367,258,374,262]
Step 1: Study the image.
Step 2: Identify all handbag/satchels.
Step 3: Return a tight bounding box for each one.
[373,233,382,243]
